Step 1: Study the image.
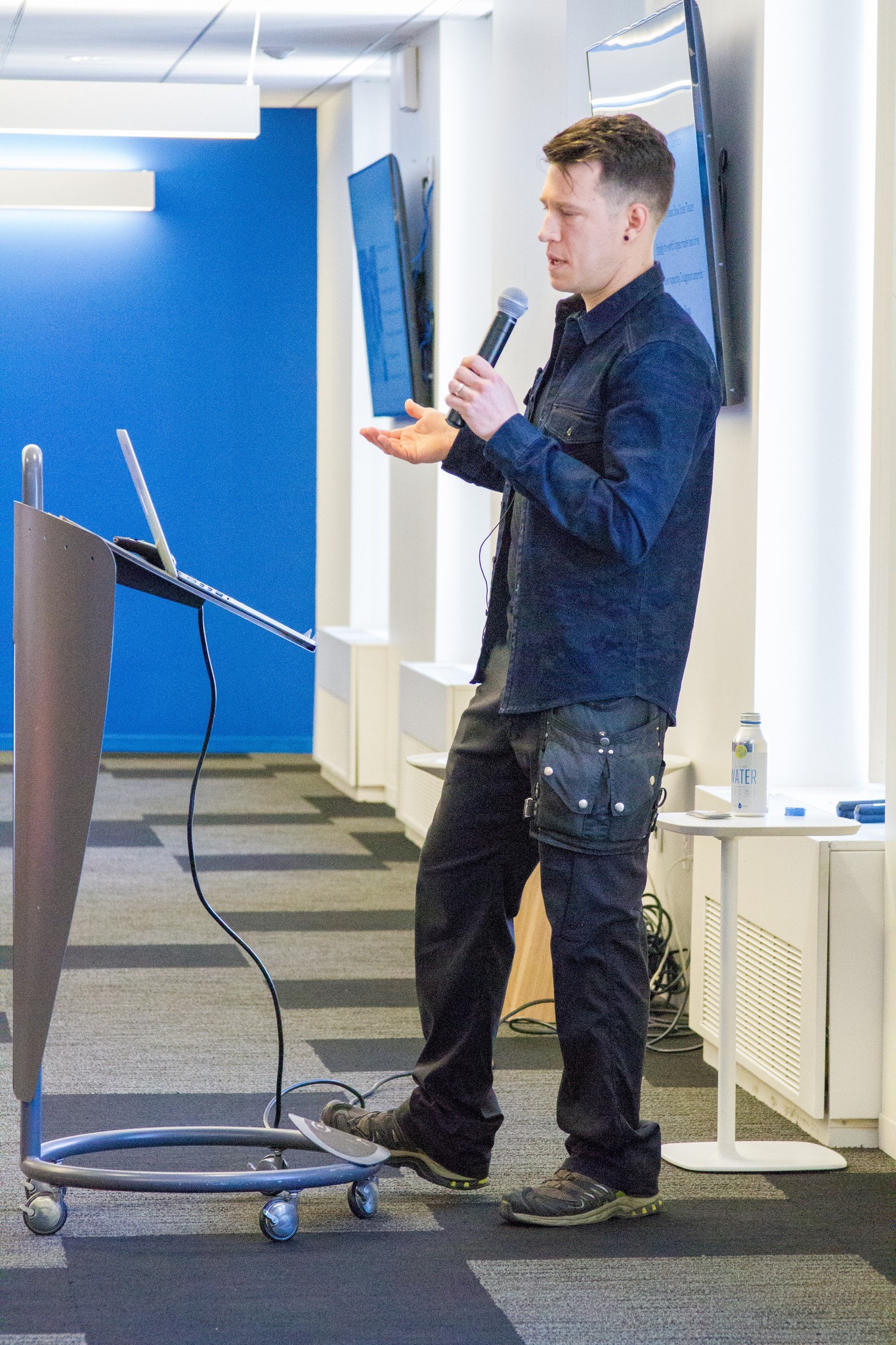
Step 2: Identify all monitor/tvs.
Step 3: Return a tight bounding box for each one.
[348,153,425,417]
[585,0,746,404]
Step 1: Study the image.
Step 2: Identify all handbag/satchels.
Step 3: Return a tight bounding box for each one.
[525,715,663,853]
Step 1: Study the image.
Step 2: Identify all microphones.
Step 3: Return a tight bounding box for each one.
[446,287,527,429]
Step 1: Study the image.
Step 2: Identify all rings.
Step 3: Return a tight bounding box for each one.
[456,383,464,397]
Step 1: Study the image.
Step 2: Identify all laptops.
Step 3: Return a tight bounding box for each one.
[115,429,316,649]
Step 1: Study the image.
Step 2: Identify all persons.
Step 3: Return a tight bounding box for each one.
[320,115,723,1225]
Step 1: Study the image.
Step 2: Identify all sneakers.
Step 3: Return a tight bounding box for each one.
[499,1165,665,1227]
[321,1098,492,1192]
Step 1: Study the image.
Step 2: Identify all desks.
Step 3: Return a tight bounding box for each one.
[652,812,862,1172]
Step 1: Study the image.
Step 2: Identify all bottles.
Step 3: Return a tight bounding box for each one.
[732,713,768,817]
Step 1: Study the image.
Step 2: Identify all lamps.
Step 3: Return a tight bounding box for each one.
[0,78,261,139]
[0,169,155,211]
